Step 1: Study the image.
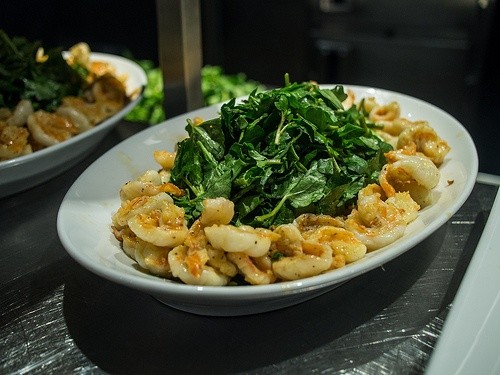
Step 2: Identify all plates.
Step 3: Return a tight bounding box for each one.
[58,84,480,317]
[0,51,147,197]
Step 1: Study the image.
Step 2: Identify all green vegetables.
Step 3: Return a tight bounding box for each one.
[165,72,394,228]
[0,30,89,114]
